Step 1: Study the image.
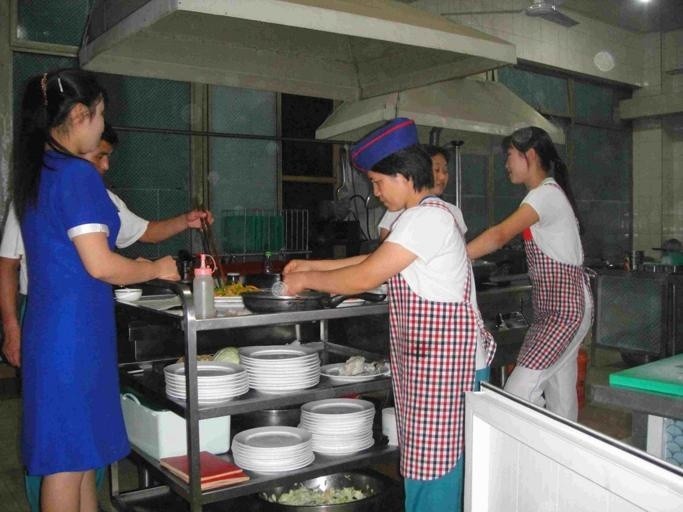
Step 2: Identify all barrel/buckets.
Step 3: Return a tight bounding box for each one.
[327,210,363,258]
[577,350,588,412]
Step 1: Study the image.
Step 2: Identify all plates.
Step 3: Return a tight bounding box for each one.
[231,425,315,475]
[238,347,323,393]
[318,363,388,381]
[299,396,376,455]
[381,407,399,440]
[213,296,245,308]
[164,362,249,406]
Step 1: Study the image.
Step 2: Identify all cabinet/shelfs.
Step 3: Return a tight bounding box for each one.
[108,288,394,512]
[474,278,532,384]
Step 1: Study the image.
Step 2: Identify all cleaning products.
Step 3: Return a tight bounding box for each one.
[623,253,631,270]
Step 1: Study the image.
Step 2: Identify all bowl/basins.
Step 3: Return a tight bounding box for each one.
[255,470,390,512]
[114,287,143,303]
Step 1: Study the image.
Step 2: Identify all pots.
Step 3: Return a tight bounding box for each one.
[473,256,509,280]
[239,287,387,314]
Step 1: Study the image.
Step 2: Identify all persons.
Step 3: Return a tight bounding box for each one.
[375,145,454,248]
[464,128,598,426]
[8,69,180,512]
[0,123,217,512]
[281,114,496,512]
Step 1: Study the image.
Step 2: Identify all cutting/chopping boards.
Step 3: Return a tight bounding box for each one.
[608,352,682,398]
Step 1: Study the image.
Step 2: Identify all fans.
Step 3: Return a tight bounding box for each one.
[440,0,580,28]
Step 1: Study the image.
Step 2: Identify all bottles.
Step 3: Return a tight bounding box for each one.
[225,272,241,287]
[192,252,219,320]
[631,250,646,272]
[262,251,274,274]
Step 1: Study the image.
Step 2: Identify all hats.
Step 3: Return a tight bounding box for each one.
[350,117,419,174]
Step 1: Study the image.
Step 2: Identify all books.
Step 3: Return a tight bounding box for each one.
[167,469,252,492]
[159,450,243,483]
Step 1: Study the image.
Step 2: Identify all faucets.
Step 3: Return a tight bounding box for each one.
[650,247,667,251]
